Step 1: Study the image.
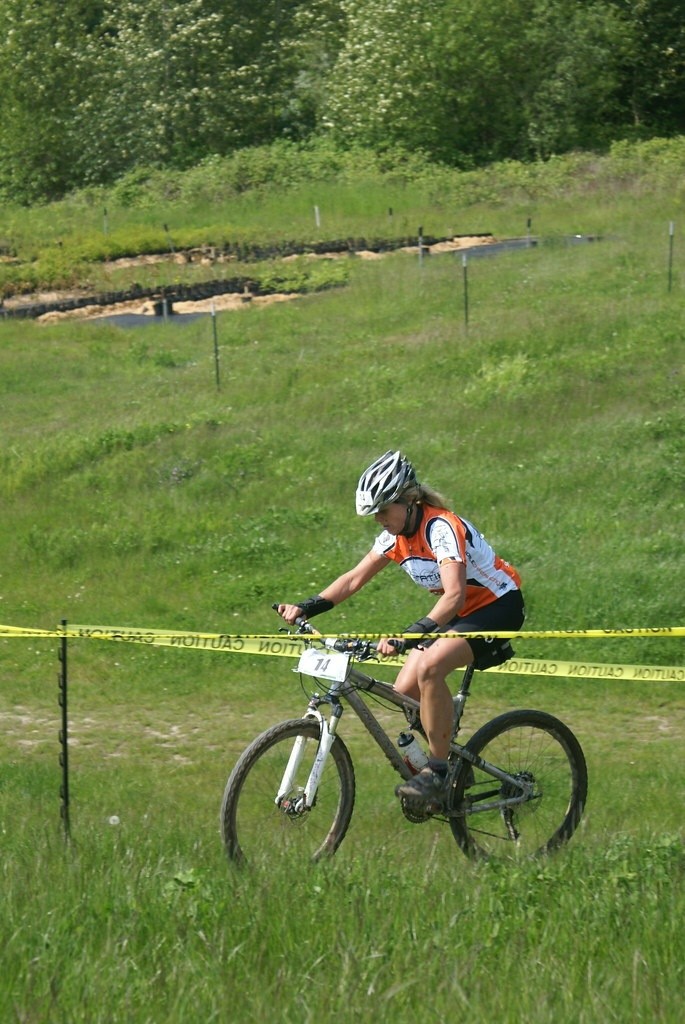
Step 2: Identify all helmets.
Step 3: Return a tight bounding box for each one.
[356,449,417,516]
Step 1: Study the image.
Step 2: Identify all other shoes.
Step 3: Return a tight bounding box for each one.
[397,760,450,801]
[464,767,475,787]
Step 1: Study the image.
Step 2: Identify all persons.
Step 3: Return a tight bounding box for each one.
[277,450,524,799]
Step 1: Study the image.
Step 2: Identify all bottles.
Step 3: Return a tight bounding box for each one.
[398,732,430,770]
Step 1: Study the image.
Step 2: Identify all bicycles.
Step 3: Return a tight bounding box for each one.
[224,604,588,867]
